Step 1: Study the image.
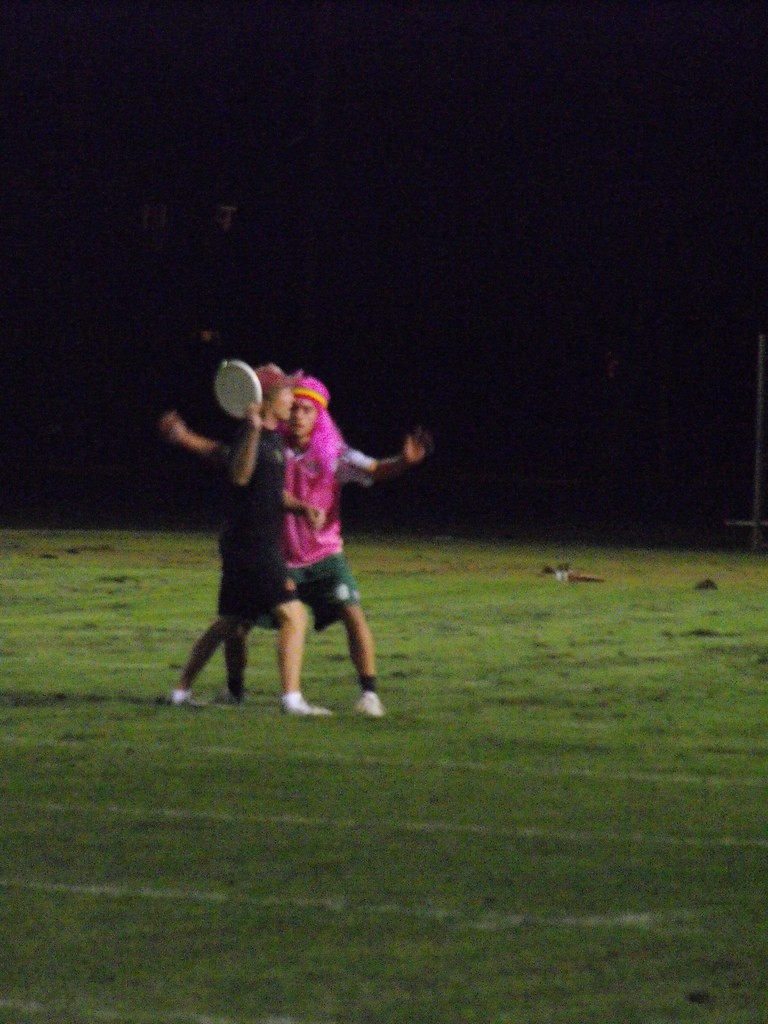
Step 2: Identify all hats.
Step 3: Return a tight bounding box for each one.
[255,364,296,393]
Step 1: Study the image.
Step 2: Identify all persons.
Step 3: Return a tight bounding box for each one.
[157,361,431,715]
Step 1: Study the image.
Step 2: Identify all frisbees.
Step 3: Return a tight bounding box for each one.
[213,358,261,420]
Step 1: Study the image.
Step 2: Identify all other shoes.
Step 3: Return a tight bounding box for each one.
[207,691,248,705]
[355,691,385,718]
[167,690,191,706]
[281,699,332,717]
